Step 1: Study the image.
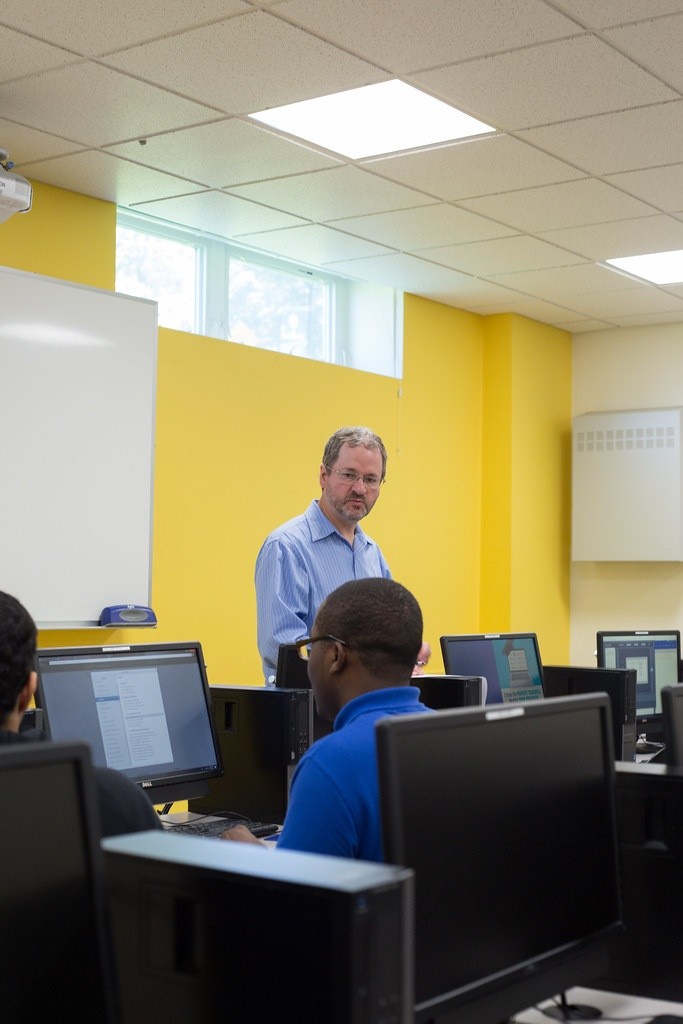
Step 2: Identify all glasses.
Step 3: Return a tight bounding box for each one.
[295,634,348,661]
[325,464,386,490]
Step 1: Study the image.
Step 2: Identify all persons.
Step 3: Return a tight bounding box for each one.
[0,589,165,834]
[220,576,434,866]
[255,429,432,693]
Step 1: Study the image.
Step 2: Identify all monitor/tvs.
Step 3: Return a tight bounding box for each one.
[440,633,545,705]
[661,683,682,765]
[0,742,106,1024]
[277,643,333,743]
[596,631,680,740]
[30,642,223,806]
[375,694,624,1024]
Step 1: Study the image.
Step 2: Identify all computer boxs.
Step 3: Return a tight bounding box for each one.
[411,676,482,711]
[577,759,683,1003]
[98,829,416,1024]
[541,666,637,764]
[187,686,313,823]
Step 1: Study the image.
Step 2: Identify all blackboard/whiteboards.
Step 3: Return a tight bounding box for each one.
[0,268,162,633]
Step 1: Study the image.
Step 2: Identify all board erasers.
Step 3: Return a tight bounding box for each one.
[99,604,158,626]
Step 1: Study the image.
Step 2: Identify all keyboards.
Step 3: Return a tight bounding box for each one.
[164,818,279,841]
